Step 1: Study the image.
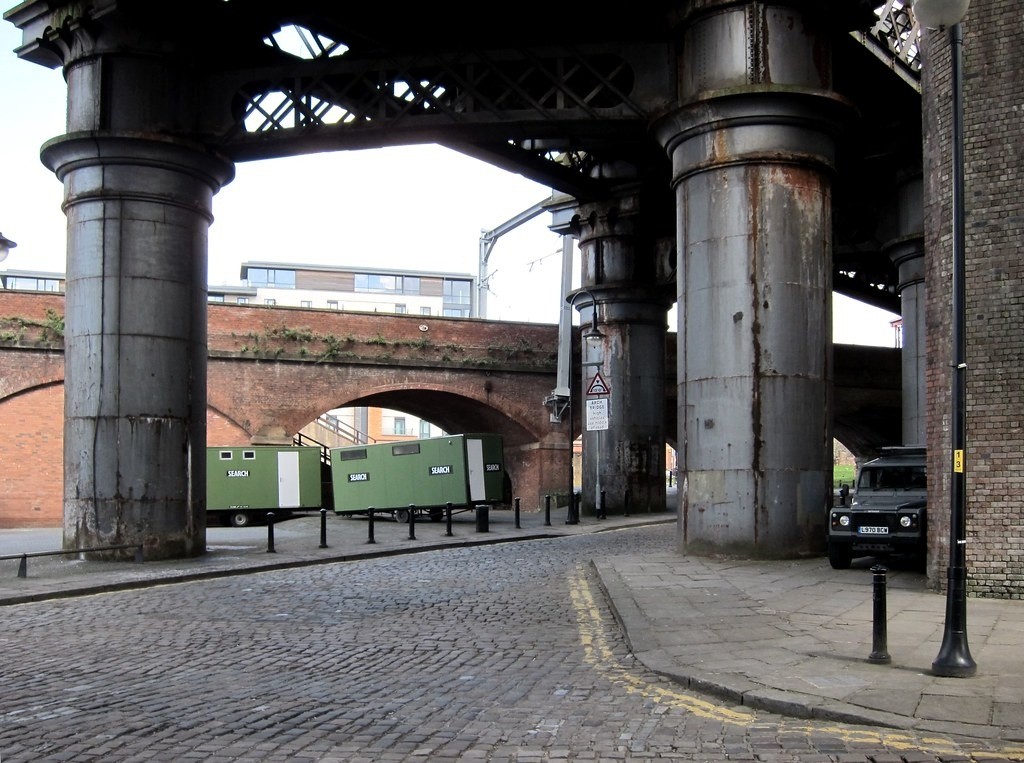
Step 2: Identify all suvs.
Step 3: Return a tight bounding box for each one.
[825,445,927,569]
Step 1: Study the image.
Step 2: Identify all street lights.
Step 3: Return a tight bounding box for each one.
[563,289,607,525]
